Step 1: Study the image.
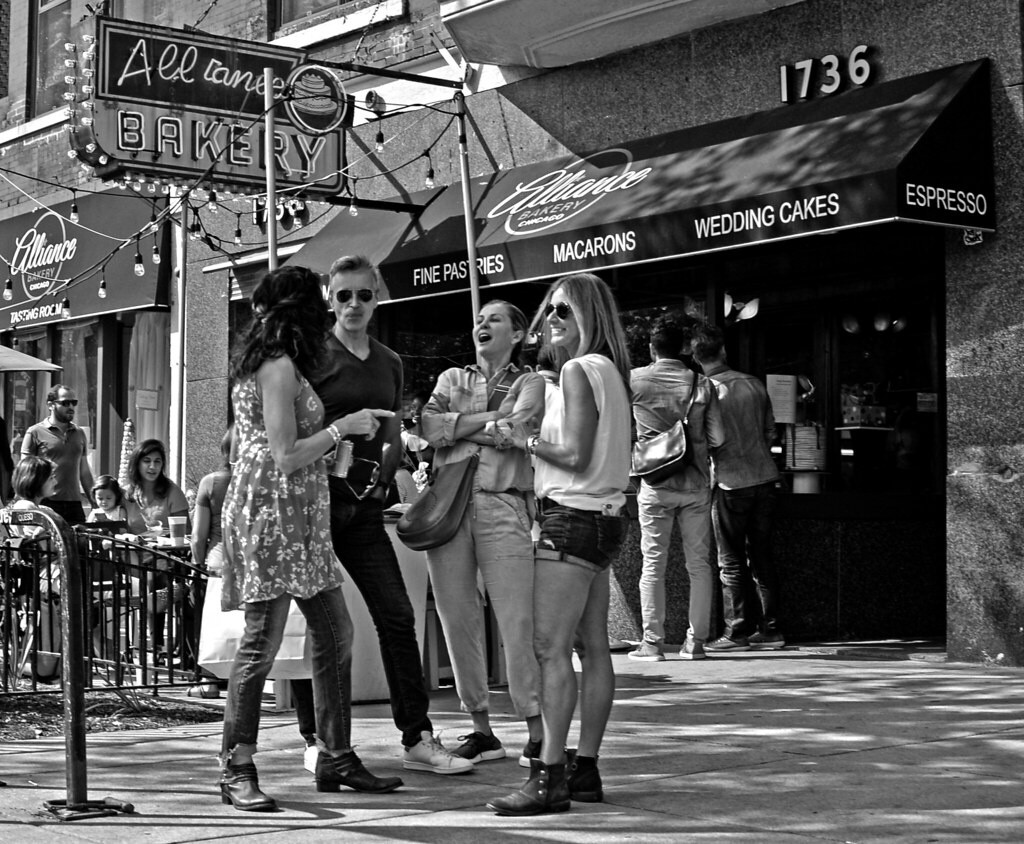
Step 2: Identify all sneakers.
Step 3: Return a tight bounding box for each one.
[302,745,319,773]
[628,643,666,662]
[450,728,506,764]
[520,739,578,768]
[679,640,705,660]
[748,630,786,649]
[402,729,473,774]
[701,635,751,652]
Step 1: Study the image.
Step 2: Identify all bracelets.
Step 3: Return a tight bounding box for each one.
[325,424,341,446]
[527,435,539,455]
[533,438,544,455]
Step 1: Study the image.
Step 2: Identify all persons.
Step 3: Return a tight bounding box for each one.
[291,254,475,775]
[186,423,234,699]
[86,474,142,524]
[0,416,15,505]
[0,455,133,671]
[123,439,192,667]
[486,273,632,817]
[628,314,723,657]
[421,301,554,768]
[689,321,783,653]
[398,394,435,490]
[20,384,103,628]
[220,266,404,812]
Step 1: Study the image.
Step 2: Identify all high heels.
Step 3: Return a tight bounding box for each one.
[314,750,403,792]
[219,763,276,811]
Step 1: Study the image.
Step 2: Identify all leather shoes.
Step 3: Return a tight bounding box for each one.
[486,758,570,815]
[568,753,604,802]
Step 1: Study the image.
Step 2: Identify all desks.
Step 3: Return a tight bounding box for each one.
[104,533,192,684]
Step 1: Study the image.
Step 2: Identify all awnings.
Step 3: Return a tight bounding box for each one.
[261,58,997,305]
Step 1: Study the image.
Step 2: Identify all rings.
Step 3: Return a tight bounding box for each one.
[373,428,377,433]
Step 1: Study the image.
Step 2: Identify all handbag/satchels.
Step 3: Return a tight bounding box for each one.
[396,456,481,551]
[200,575,317,680]
[630,421,688,486]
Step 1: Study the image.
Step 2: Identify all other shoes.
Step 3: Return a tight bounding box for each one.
[152,654,166,666]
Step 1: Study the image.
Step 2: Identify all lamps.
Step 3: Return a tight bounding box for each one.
[725,292,759,321]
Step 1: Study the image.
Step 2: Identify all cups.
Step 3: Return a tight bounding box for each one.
[167,516,187,548]
[330,441,354,478]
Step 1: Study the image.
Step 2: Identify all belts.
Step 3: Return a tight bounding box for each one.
[534,495,559,515]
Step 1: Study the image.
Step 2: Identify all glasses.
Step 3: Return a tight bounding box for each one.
[545,302,573,320]
[51,399,79,408]
[346,456,381,500]
[333,288,374,303]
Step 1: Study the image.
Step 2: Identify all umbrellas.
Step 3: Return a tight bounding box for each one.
[0,344,64,371]
